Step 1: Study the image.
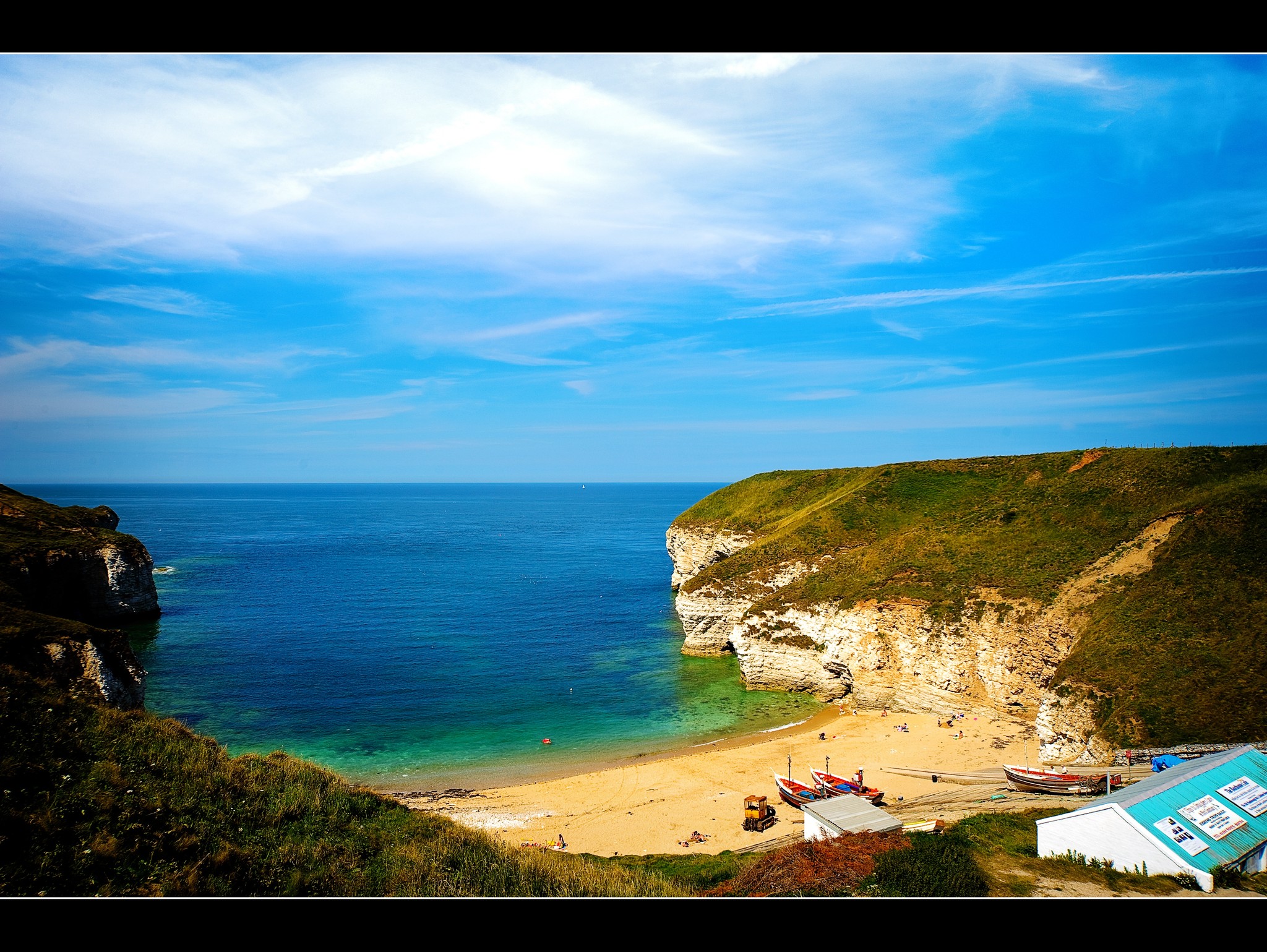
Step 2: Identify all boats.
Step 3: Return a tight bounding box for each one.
[807,755,885,805]
[153,567,168,573]
[1002,739,1123,795]
[770,744,830,807]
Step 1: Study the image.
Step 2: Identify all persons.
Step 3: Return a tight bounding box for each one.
[819,706,857,740]
[881,709,908,732]
[696,833,706,843]
[678,839,688,847]
[938,717,940,726]
[948,734,957,736]
[957,730,963,739]
[952,712,964,720]
[690,831,698,839]
[521,841,542,848]
[852,778,856,783]
[946,720,952,726]
[558,833,568,848]
[1051,766,1055,771]
[1061,765,1067,774]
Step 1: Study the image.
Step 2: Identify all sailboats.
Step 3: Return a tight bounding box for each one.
[581,485,586,489]
